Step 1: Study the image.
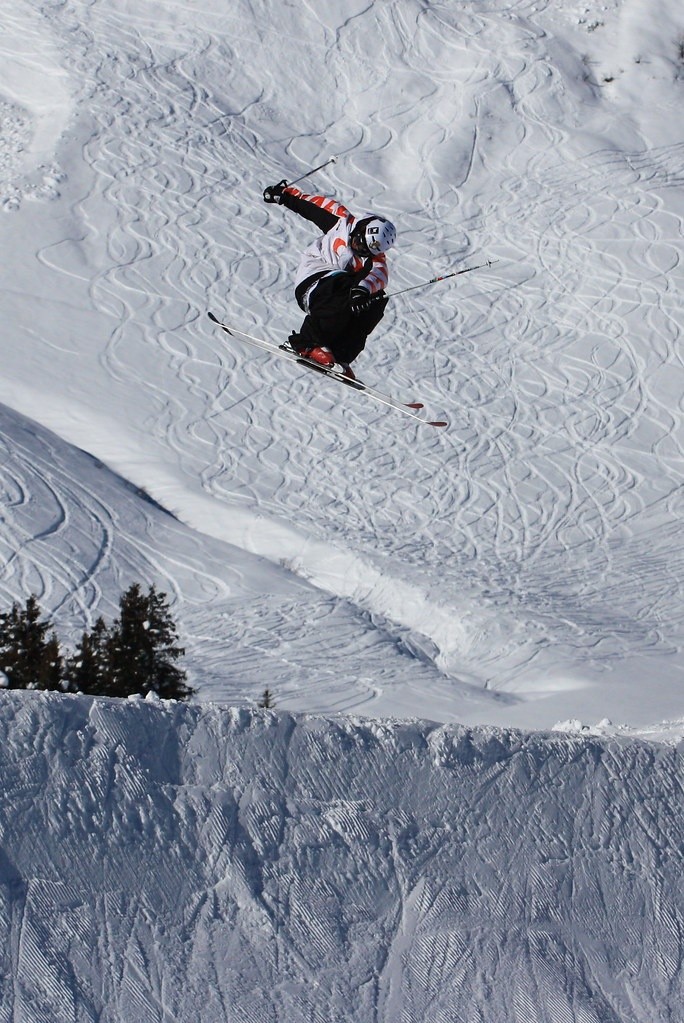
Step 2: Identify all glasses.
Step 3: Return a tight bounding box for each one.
[355,234,370,259]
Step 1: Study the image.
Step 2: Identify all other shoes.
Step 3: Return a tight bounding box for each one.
[342,362,356,378]
[299,345,335,367]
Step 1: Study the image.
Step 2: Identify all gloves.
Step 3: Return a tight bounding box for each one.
[349,286,372,315]
[263,185,287,205]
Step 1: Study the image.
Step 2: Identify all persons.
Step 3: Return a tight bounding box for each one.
[263,184,397,379]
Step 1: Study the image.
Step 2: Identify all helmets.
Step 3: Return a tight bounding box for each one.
[351,215,396,259]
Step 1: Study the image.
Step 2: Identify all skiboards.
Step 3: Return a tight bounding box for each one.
[206,311,447,428]
